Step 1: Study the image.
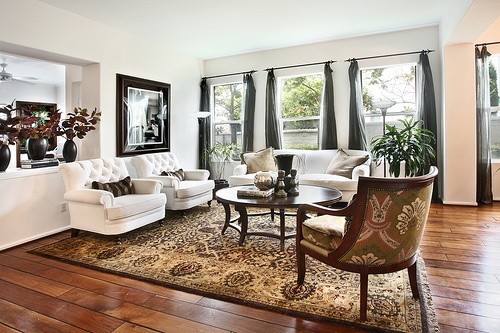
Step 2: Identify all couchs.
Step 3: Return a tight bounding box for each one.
[228,149,371,204]
[130,151,215,217]
[60,159,167,244]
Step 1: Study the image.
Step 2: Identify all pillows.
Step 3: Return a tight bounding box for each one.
[326,149,369,179]
[91,176,134,197]
[160,168,185,182]
[244,147,275,173]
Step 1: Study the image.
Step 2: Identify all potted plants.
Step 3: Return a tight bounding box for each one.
[202,143,238,200]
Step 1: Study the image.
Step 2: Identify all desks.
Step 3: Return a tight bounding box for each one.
[215,183,343,253]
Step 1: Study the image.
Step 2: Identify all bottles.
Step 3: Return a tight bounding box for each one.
[288,169,299,196]
[275,170,288,198]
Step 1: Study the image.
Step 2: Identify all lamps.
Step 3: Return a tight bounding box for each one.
[195,111,211,169]
[373,101,397,178]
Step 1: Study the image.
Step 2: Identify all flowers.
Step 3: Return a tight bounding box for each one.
[0,98,102,143]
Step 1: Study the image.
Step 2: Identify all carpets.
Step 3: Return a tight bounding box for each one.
[26,200,440,333]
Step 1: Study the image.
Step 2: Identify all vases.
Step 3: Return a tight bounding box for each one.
[26,138,48,161]
[0,143,11,170]
[63,140,78,163]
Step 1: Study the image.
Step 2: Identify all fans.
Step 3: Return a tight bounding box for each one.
[0,63,34,83]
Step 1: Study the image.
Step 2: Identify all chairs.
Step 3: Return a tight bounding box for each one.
[295,166,438,323]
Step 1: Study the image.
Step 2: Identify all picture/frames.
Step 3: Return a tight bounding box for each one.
[116,74,171,156]
